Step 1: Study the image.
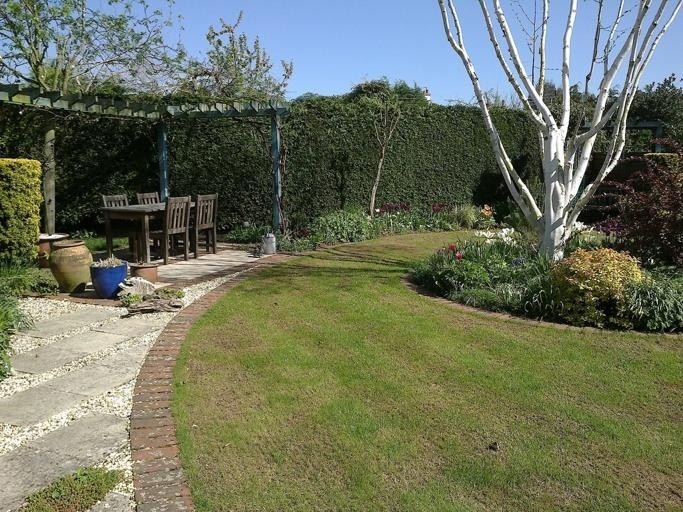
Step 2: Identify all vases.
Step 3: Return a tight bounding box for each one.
[88,261,160,299]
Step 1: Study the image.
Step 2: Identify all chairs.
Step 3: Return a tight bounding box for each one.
[97,190,219,266]
